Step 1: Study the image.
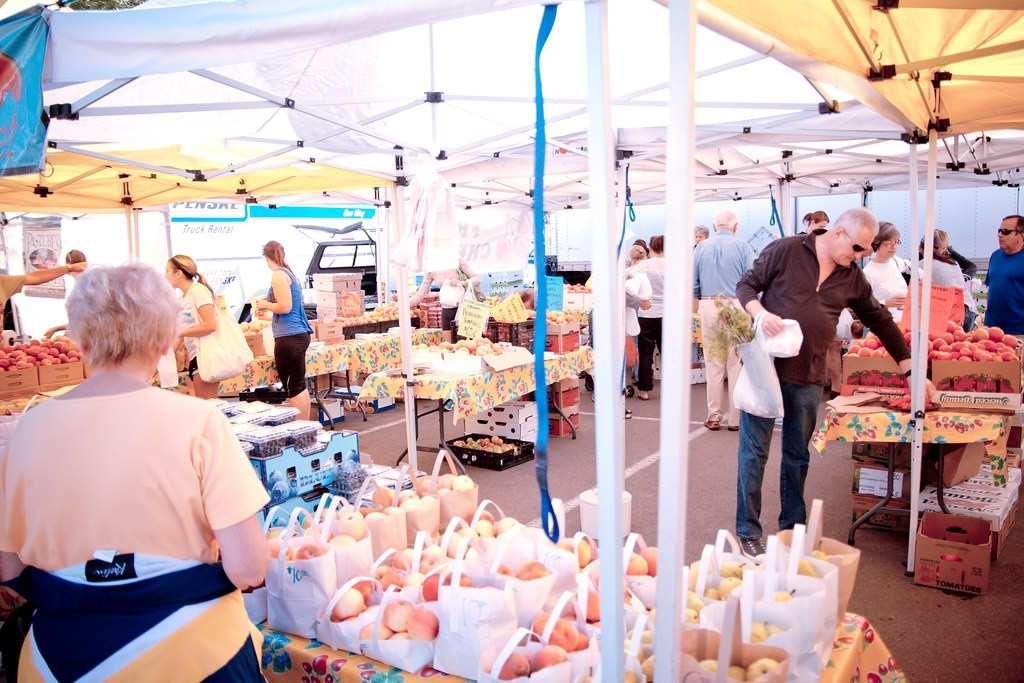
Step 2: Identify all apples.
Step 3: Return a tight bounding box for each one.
[848,321,1019,362]
[567,283,588,292]
[454,435,516,453]
[0,335,81,371]
[268,474,826,683]
[240,320,272,335]
[308,300,417,326]
[546,311,575,325]
[412,337,505,355]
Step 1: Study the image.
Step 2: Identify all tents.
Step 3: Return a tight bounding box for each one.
[2,3,1022,682]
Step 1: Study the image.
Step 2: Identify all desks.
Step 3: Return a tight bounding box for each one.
[815,408,1013,575]
[218,346,367,429]
[253,607,910,683]
[359,346,595,475]
[341,327,453,375]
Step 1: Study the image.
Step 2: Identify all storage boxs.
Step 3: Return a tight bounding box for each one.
[843,339,1024,597]
[0,274,580,530]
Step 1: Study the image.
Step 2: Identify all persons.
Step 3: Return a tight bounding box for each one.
[0,250,88,349]
[857,220,980,340]
[985,215,1024,336]
[409,253,482,335]
[736,206,935,565]
[691,211,756,431]
[254,240,313,421]
[626,225,664,418]
[0,263,272,683]
[163,255,226,402]
[796,211,830,236]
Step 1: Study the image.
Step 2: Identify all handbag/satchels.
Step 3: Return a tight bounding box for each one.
[440,279,465,309]
[455,281,480,326]
[190,284,255,382]
[733,311,785,418]
[624,273,652,302]
[261,450,862,683]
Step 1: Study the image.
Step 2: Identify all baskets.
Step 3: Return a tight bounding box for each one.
[246,430,359,530]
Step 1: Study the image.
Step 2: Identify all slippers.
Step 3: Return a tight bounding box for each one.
[638,393,649,400]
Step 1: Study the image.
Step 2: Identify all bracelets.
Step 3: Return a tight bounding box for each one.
[68,264,73,273]
[904,370,912,378]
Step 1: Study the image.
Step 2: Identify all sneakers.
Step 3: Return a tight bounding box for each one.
[738,536,767,564]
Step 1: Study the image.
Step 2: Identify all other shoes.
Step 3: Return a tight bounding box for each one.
[705,420,720,430]
[728,425,739,431]
[625,408,632,419]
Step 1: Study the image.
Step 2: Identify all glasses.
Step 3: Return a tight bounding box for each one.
[998,228,1018,236]
[880,239,901,248]
[843,231,868,252]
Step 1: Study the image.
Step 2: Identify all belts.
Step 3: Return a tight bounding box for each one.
[701,297,738,300]
